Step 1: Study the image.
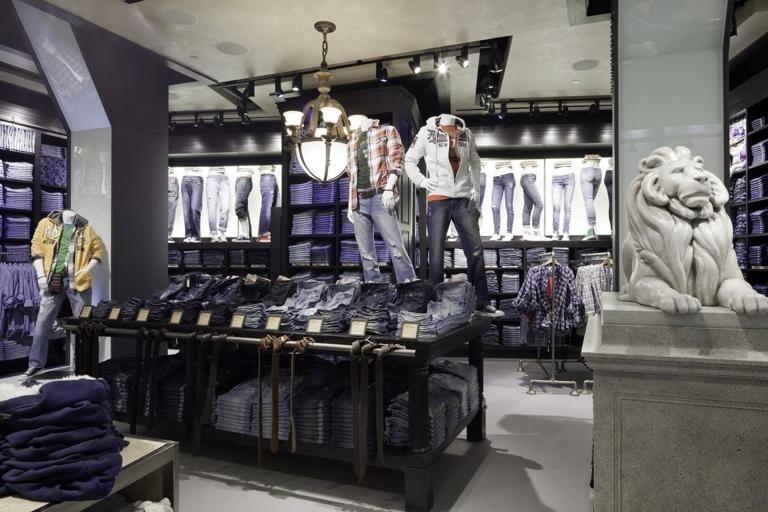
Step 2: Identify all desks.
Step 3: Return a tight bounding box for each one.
[0,432,185,510]
[54,313,493,510]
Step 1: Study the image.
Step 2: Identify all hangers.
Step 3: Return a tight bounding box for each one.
[0,253,33,265]
[535,253,613,267]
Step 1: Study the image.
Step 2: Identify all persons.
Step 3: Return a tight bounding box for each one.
[16,210,107,380]
[404,113,490,311]
[168,166,280,239]
[345,117,418,282]
[478,157,615,244]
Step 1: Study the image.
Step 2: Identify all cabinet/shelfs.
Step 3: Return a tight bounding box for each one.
[1,122,71,366]
[166,244,272,276]
[422,244,615,355]
[283,110,403,279]
[728,95,768,296]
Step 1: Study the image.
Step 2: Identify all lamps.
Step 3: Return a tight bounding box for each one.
[292,17,354,184]
[496,102,601,120]
[271,75,303,99]
[378,43,473,85]
[480,58,504,111]
[186,105,256,131]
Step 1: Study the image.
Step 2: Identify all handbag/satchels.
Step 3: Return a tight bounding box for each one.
[48,272,64,294]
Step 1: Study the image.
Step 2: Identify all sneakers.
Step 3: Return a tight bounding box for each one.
[219,235,228,243]
[581,228,597,241]
[232,235,252,242]
[183,237,192,243]
[490,235,501,241]
[473,304,507,318]
[551,233,560,241]
[561,233,570,241]
[211,235,218,243]
[189,237,202,243]
[23,367,43,377]
[168,238,175,243]
[502,234,513,242]
[259,235,272,242]
